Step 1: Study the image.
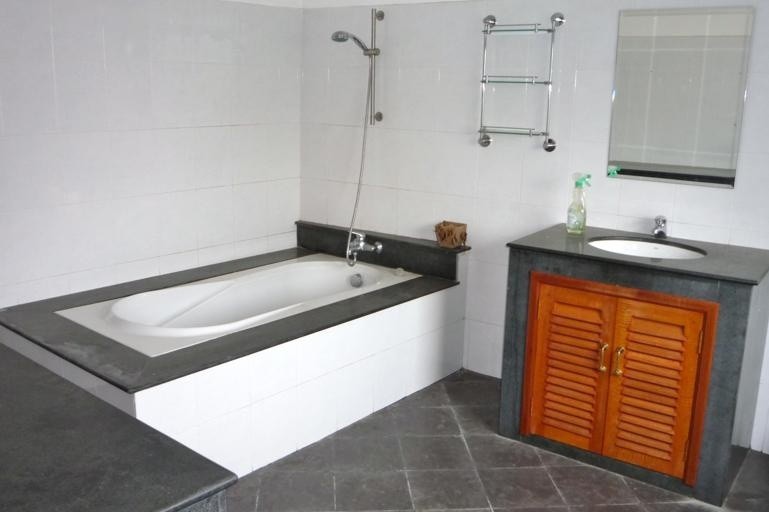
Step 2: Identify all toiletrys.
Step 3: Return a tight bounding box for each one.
[607,164,623,177]
[565,173,594,235]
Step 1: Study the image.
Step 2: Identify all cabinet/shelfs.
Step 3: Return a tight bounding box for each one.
[476,12,567,153]
[519,268,720,488]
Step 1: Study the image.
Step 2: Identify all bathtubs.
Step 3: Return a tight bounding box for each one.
[53,246,424,359]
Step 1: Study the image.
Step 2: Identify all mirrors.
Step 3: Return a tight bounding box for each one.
[607,4,759,190]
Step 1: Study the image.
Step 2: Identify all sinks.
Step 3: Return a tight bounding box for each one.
[585,234,709,261]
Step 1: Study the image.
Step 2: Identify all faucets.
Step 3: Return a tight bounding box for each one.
[347,231,384,267]
[653,215,668,237]
